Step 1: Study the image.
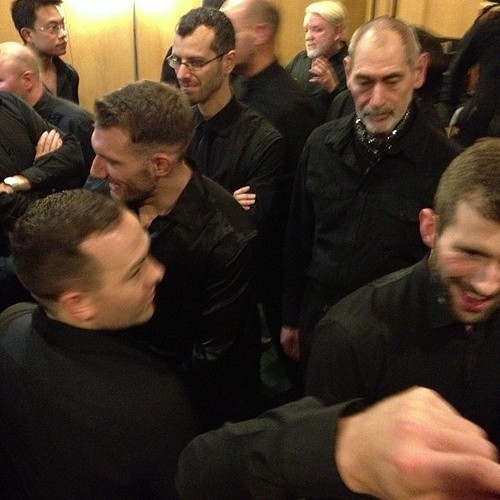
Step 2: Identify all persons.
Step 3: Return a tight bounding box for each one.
[81,79,261,434]
[0,189,197,500]
[304,137,500,448]
[0,0,500,308]
[174,385,500,500]
[0,90,86,313]
[279,16,469,400]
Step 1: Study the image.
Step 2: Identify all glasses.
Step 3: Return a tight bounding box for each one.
[28,22,65,33]
[166,52,231,71]
